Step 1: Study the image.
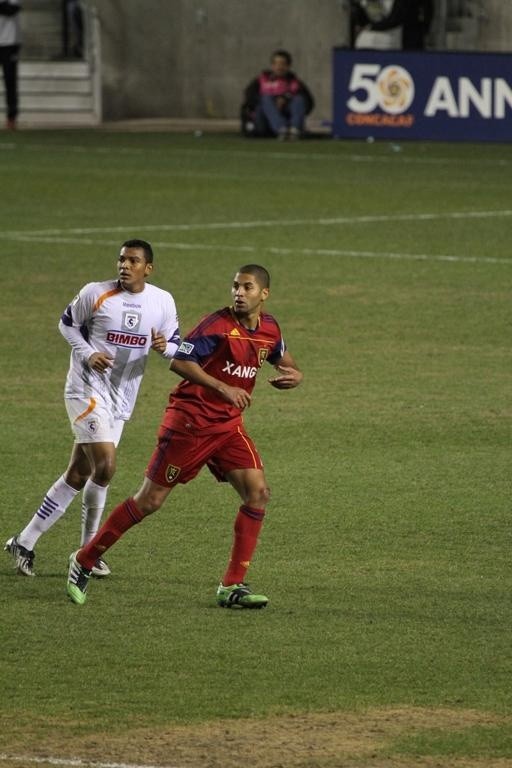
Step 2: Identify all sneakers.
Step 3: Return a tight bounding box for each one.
[214,579,270,607]
[67,549,89,606]
[68,546,110,579]
[5,536,35,576]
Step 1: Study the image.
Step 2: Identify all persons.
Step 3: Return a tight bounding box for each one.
[349,0,438,53]
[0,0,24,135]
[3,237,180,582]
[62,263,305,613]
[235,50,315,143]
